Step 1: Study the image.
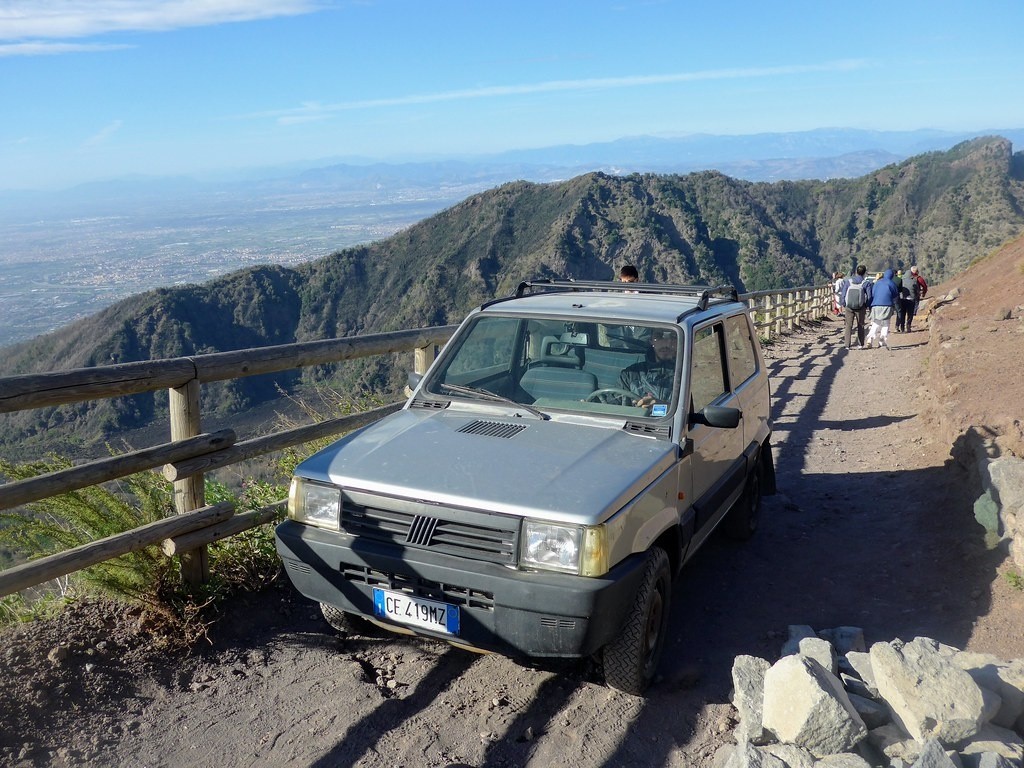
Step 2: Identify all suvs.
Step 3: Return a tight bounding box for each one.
[275,277,781,699]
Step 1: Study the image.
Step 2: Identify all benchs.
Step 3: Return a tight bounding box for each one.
[548,343,648,391]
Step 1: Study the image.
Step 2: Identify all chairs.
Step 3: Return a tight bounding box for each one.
[513,354,597,406]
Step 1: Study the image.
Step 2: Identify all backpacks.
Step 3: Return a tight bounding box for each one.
[844,279,868,310]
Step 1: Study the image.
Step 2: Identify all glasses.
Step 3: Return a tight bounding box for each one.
[622,278,637,282]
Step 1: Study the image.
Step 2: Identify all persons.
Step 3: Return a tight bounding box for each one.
[620,265,639,294]
[580,326,677,409]
[827,265,927,350]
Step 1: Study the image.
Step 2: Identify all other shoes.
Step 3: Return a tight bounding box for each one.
[878,341,886,349]
[866,342,873,349]
[855,345,863,350]
[893,330,900,333]
[907,329,911,333]
[901,329,904,332]
[843,346,849,351]
